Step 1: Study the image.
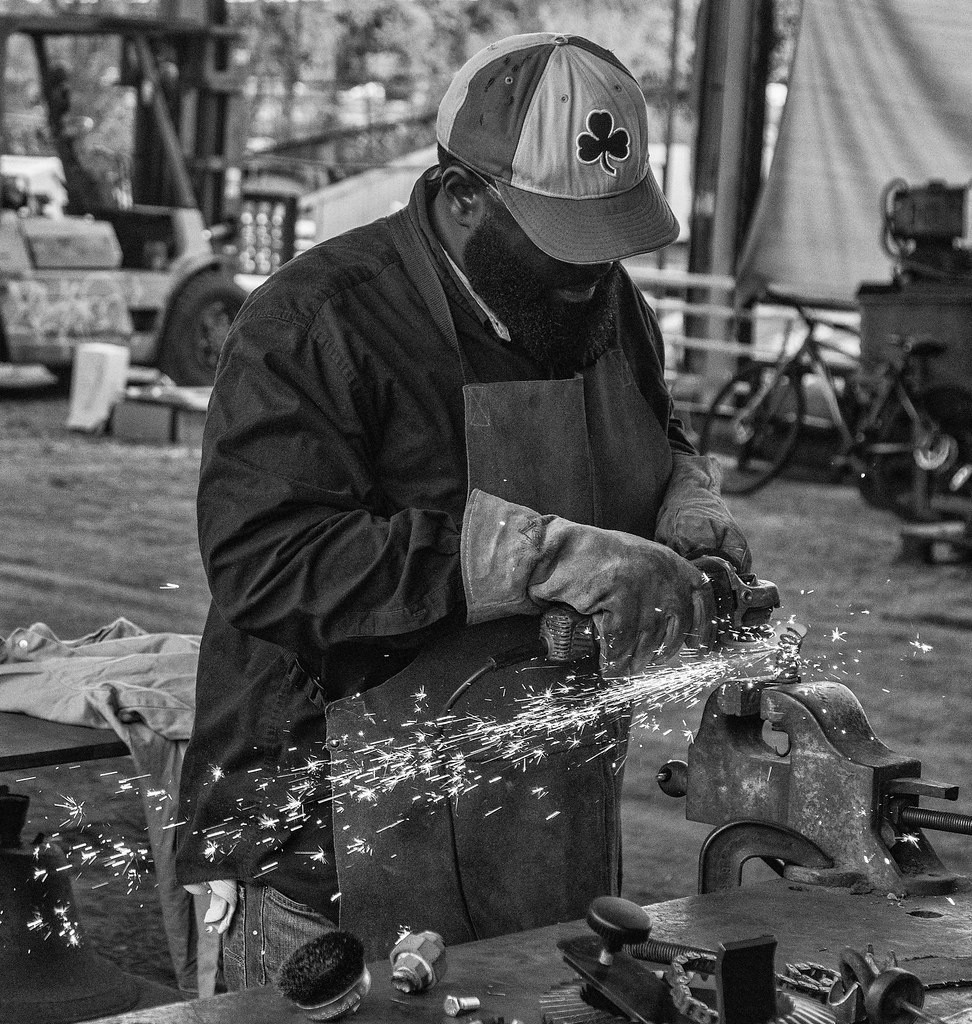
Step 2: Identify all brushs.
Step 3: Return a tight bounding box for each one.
[271,930,371,1019]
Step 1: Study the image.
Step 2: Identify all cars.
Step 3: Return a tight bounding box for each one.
[0,151,249,387]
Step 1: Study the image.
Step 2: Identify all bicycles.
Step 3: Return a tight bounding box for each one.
[694,289,972,520]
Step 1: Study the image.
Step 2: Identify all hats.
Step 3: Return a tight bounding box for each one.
[436,32,680,265]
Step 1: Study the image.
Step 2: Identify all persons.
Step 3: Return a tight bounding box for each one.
[173,32,752,1000]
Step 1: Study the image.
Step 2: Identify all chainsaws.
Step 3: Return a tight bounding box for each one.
[540,551,787,691]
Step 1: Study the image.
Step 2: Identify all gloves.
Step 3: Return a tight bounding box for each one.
[460,488,718,680]
[654,456,752,575]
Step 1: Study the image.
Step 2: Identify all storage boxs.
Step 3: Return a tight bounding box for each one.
[67,342,209,444]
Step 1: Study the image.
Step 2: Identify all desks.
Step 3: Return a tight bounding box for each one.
[0,681,972,1024]
